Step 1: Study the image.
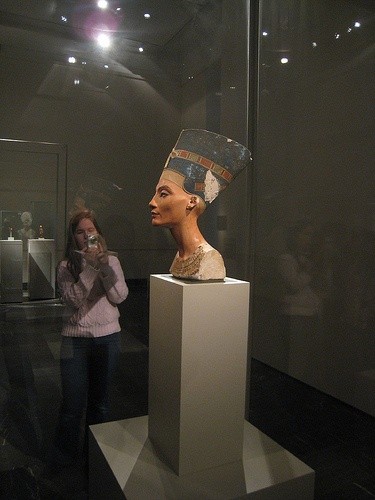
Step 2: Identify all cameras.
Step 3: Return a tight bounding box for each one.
[88,235,97,249]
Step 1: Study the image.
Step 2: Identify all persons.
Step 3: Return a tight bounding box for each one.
[150,129,252,283]
[67,184,119,252]
[56,211,129,455]
[17,211,36,240]
